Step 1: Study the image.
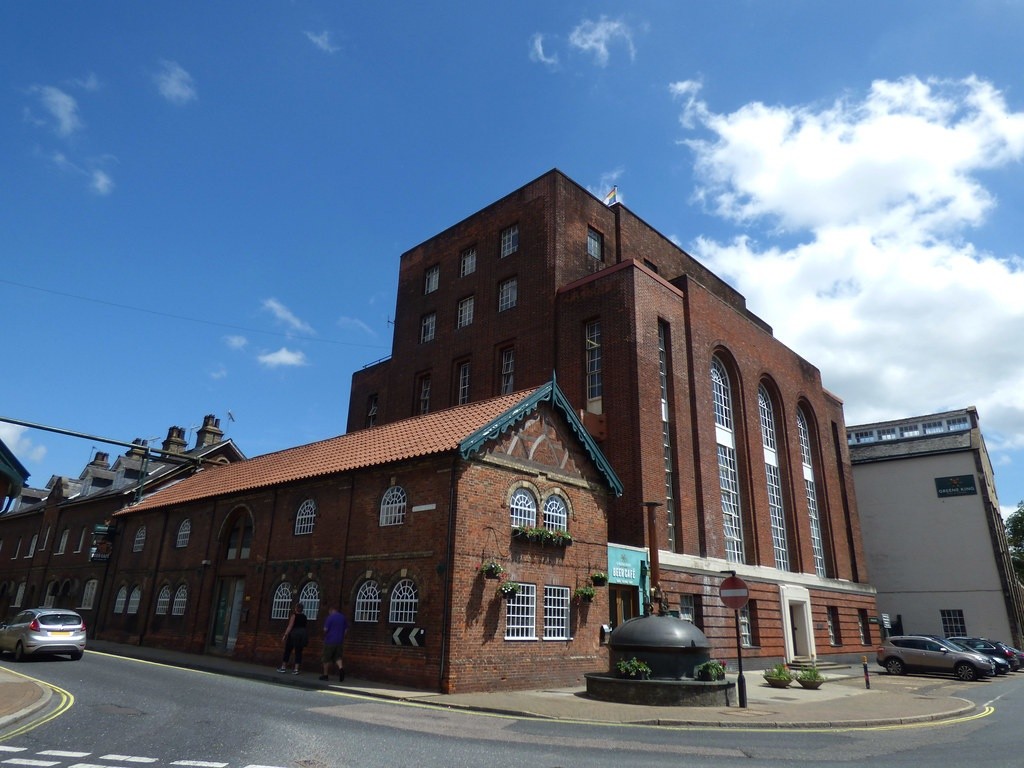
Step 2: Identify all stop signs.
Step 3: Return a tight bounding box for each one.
[718,576,750,609]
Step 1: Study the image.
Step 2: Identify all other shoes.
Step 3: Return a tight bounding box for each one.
[319,675,329,680]
[276,667,286,673]
[338,668,345,682]
[291,670,299,674]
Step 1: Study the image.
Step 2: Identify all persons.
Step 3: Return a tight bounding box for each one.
[321,605,347,681]
[276,603,306,674]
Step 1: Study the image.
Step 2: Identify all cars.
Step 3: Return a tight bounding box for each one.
[876,635,995,681]
[0,608,87,663]
[908,634,1024,675]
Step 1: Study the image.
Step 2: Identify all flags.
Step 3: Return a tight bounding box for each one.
[603,189,617,206]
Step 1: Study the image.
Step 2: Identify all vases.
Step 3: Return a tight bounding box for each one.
[583,594,593,602]
[485,570,501,580]
[717,673,726,680]
[592,578,607,586]
[504,592,516,599]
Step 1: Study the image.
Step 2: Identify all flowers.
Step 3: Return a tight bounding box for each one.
[501,581,521,593]
[588,571,608,581]
[716,659,728,673]
[473,562,507,580]
[573,586,597,605]
[511,526,574,545]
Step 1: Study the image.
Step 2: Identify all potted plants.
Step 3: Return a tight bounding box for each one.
[697,661,720,681]
[612,657,651,679]
[795,663,825,689]
[763,663,795,688]
